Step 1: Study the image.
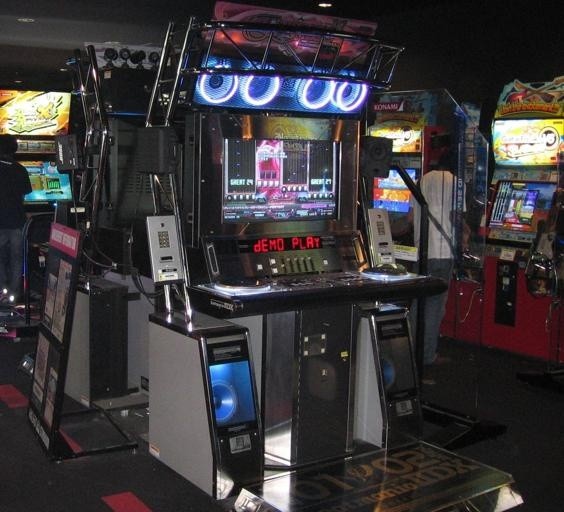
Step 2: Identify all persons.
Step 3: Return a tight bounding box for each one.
[406,146,471,367]
[0,134,32,308]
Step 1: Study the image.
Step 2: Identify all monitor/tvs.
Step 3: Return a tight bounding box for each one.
[222,137,340,224]
[488,180,558,234]
[16,159,73,202]
[371,167,417,214]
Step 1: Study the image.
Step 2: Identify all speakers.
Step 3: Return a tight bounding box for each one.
[149,309,264,501]
[185,49,374,120]
[355,303,423,449]
